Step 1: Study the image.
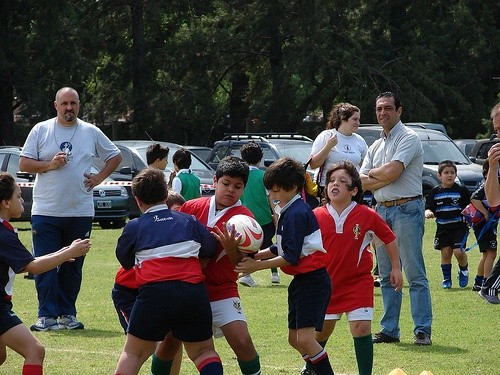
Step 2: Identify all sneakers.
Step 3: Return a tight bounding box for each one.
[239,274,256,287]
[459,269,468,287]
[443,278,451,288]
[373,332,400,343]
[473,285,481,291]
[272,274,280,283]
[478,291,500,304]
[414,332,432,345]
[58,315,84,329]
[30,316,59,331]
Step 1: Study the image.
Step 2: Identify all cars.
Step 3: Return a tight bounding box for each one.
[404,122,500,166]
[93,141,216,221]
[0,145,129,230]
[182,145,221,163]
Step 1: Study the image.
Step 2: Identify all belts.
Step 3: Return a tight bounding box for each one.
[380,196,421,206]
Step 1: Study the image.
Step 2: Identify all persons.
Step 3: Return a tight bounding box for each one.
[145,143,170,171]
[172,148,201,202]
[358,91,433,345]
[470,158,500,291]
[0,173,92,375]
[233,158,334,375]
[111,192,186,335]
[151,156,261,375]
[310,103,368,199]
[112,169,223,375]
[301,161,404,375]
[19,87,123,331]
[482,102,500,207]
[479,257,500,304]
[425,161,474,287]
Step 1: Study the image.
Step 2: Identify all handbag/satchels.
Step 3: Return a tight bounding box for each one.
[303,157,324,209]
[461,199,489,228]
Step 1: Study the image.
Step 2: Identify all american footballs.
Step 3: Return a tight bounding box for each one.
[226,215,264,253]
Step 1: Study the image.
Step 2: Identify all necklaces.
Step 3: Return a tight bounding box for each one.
[54,123,78,153]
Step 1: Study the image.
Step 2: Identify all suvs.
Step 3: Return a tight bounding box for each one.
[205,133,319,183]
[353,122,486,210]
